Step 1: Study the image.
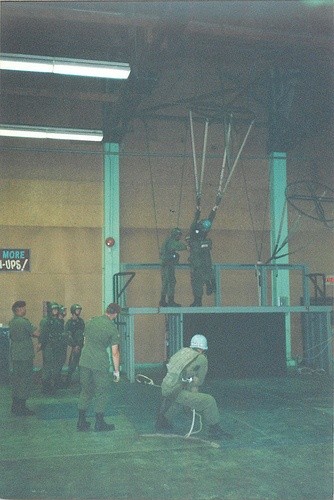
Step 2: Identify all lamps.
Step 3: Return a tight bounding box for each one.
[0,123,104,142]
[0,53,131,80]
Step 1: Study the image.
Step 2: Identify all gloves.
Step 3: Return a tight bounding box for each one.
[113,371,121,384]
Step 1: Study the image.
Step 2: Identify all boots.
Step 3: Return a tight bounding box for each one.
[11,397,34,416]
[167,295,181,307]
[158,295,168,307]
[95,412,115,433]
[190,295,202,307]
[76,411,92,431]
[206,280,217,296]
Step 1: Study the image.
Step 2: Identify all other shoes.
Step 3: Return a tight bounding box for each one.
[156,413,171,429]
[208,424,233,440]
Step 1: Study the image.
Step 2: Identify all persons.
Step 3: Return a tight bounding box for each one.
[37,302,85,394]
[189,205,219,307]
[154,334,233,440]
[8,301,40,416]
[77,302,122,431]
[159,227,189,307]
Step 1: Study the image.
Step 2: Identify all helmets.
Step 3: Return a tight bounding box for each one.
[47,302,59,313]
[190,334,209,351]
[58,305,68,315]
[199,219,211,232]
[71,304,82,315]
[171,227,182,236]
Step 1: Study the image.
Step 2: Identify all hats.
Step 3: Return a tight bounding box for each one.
[111,303,121,313]
